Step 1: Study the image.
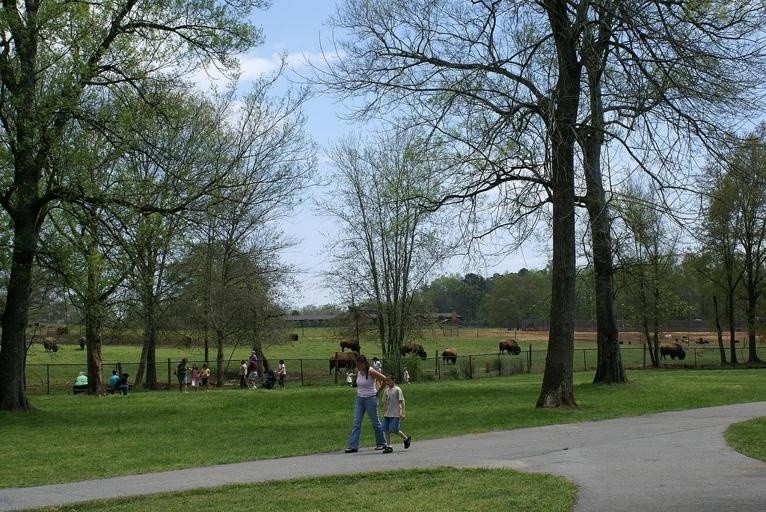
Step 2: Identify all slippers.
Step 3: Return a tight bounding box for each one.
[404,436,411,448]
[345,448,357,452]
[383,446,393,453]
[375,445,386,450]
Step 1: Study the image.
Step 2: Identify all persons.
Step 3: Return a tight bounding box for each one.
[403,366,409,385]
[238,349,288,390]
[175,357,190,393]
[200,362,210,392]
[114,373,129,395]
[344,354,387,452]
[73,371,88,385]
[345,357,383,386]
[382,375,411,454]
[110,369,119,385]
[190,364,201,392]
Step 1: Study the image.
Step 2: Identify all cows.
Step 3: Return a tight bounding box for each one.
[340,340,361,353]
[403,343,427,361]
[79,336,86,350]
[442,349,457,364]
[185,332,192,349]
[660,342,686,361]
[329,352,359,375]
[498,338,521,356]
[43,337,58,353]
